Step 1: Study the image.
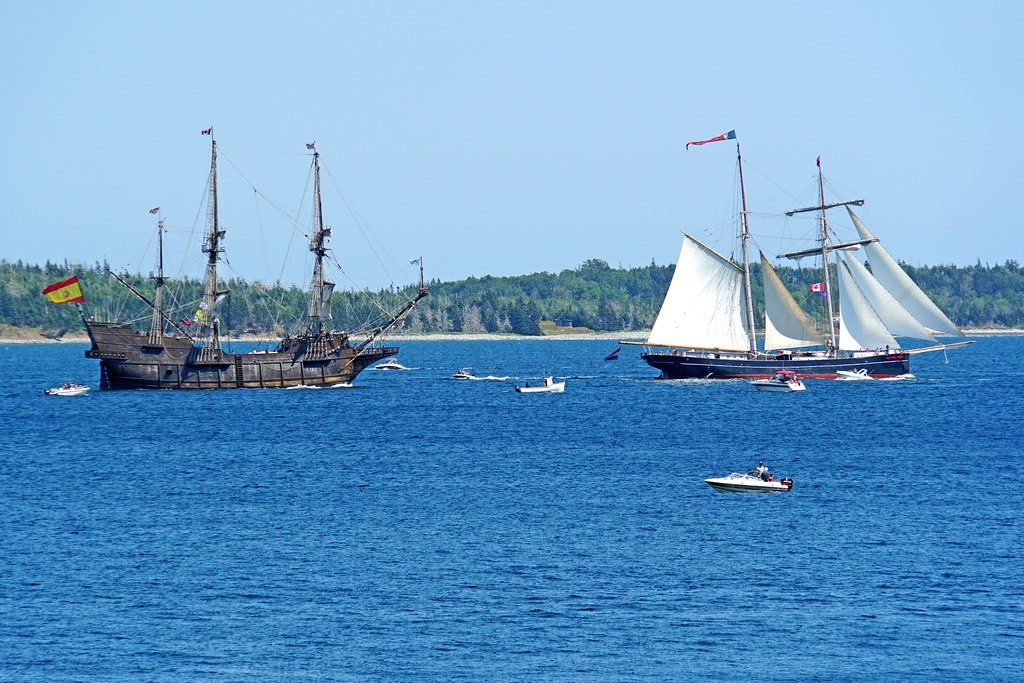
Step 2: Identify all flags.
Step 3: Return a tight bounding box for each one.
[202,129,210,136]
[42,274,85,304]
[149,206,159,214]
[810,282,824,293]
[306,143,315,149]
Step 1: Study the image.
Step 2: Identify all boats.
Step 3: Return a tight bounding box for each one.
[376,357,408,370]
[454,370,475,379]
[833,368,874,382]
[705,472,789,493]
[514,377,566,395]
[750,370,807,394]
[44,384,90,397]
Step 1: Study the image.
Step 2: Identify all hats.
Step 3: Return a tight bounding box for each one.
[759,461,763,464]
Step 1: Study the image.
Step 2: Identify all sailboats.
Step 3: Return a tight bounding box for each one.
[621,132,977,378]
[72,126,430,391]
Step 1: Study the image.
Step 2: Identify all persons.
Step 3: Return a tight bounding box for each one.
[62,381,78,389]
[757,460,776,482]
